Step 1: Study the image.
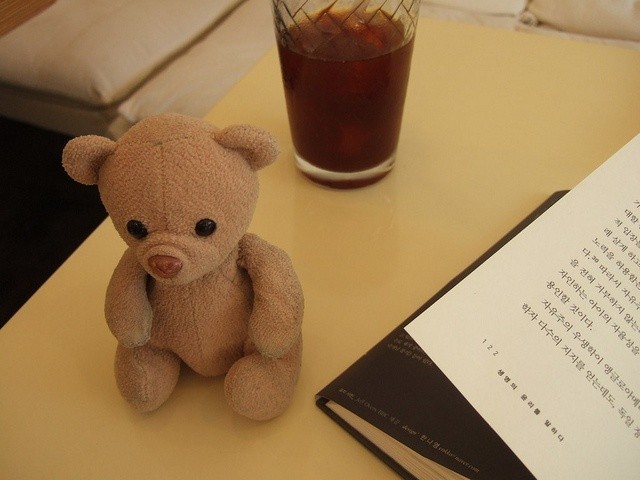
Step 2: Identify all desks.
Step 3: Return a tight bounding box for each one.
[0,9,640,479]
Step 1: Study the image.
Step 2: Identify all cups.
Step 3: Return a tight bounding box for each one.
[269,1,422,191]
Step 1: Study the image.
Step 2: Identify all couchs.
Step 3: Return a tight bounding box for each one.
[1,1,639,143]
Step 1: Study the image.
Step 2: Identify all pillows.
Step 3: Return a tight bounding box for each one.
[1,1,242,107]
[116,1,277,125]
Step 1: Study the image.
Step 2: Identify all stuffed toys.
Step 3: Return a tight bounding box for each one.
[62,111,303,422]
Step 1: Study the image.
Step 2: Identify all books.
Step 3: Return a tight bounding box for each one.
[313,187,640,480]
